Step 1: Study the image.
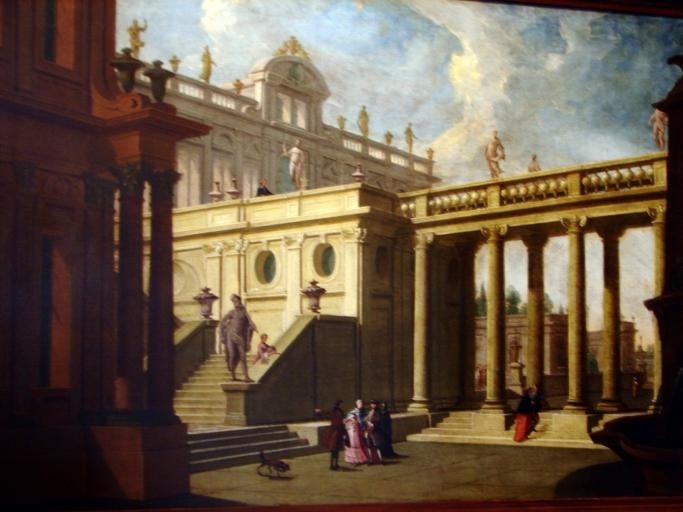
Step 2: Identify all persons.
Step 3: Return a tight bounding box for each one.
[512,386,535,438]
[324,392,396,468]
[404,120,417,152]
[486,130,504,179]
[648,108,668,152]
[252,330,274,361]
[219,291,258,382]
[527,380,539,425]
[280,136,305,189]
[255,177,274,195]
[358,105,369,133]
[196,45,217,80]
[127,16,147,56]
